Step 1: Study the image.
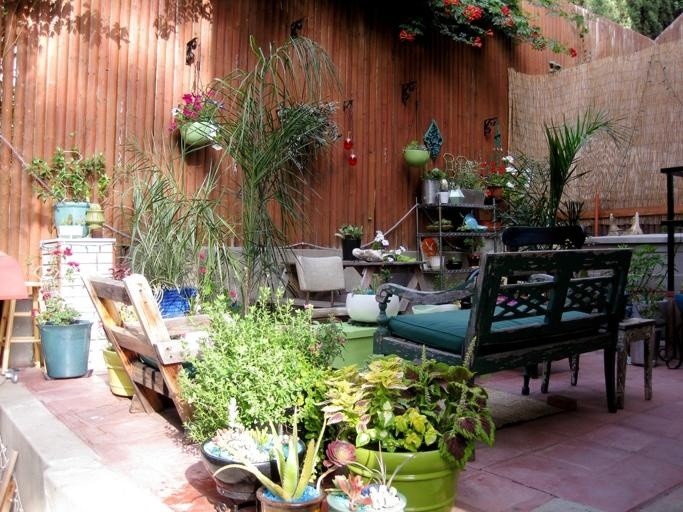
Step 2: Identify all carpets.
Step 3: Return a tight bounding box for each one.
[463,386,563,429]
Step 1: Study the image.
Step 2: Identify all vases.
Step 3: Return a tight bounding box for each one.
[486,187,504,200]
[181,123,218,147]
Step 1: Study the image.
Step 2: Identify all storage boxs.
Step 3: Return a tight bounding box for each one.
[310,323,378,370]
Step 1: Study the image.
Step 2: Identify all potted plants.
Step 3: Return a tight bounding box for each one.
[426,217,453,232]
[421,165,486,205]
[335,223,364,260]
[347,270,400,323]
[103,345,136,397]
[464,236,485,267]
[402,140,431,167]
[200,338,495,512]
[29,148,109,237]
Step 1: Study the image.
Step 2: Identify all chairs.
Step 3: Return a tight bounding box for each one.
[462,225,585,307]
[80,275,240,429]
[297,255,347,318]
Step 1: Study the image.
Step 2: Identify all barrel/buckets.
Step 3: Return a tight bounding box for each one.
[421,179,441,203]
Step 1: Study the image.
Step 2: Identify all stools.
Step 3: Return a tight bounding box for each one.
[1,281,41,373]
[542,317,655,410]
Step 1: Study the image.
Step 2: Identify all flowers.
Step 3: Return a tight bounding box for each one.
[480,158,509,185]
[175,288,348,440]
[29,247,84,326]
[169,90,220,131]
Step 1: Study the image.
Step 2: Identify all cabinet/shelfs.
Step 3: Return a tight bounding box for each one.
[416,197,498,292]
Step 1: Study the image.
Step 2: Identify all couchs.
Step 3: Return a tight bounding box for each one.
[373,250,631,414]
